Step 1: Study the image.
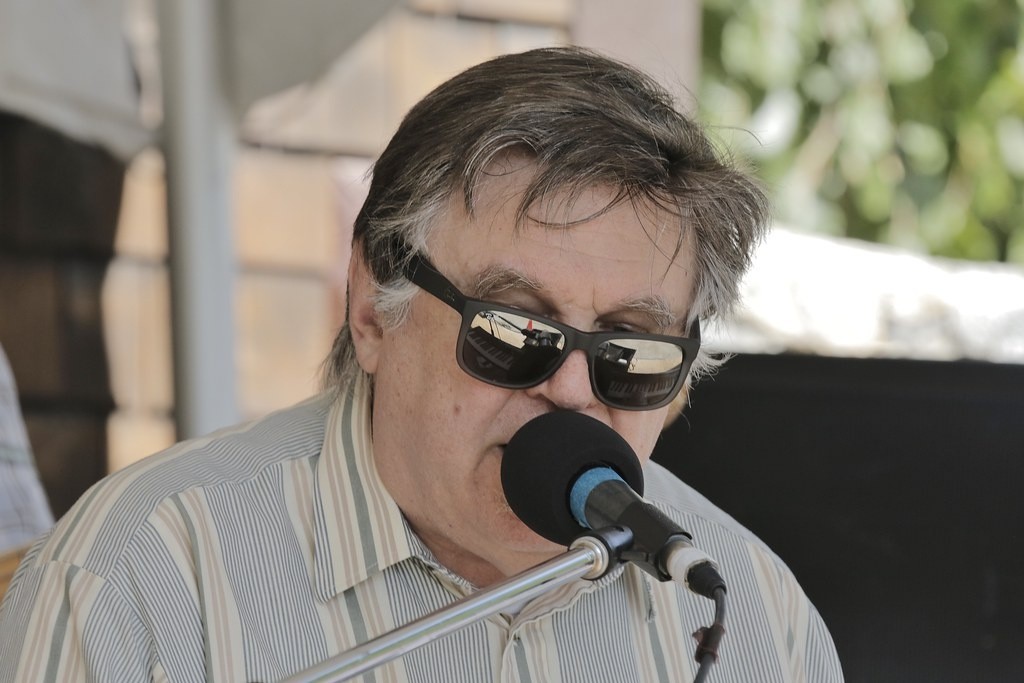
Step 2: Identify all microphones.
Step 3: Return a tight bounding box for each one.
[500,408,727,598]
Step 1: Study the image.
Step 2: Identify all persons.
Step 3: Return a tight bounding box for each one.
[0,44,847,683]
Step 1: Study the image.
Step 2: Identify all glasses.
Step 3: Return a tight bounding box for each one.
[389,232,702,410]
